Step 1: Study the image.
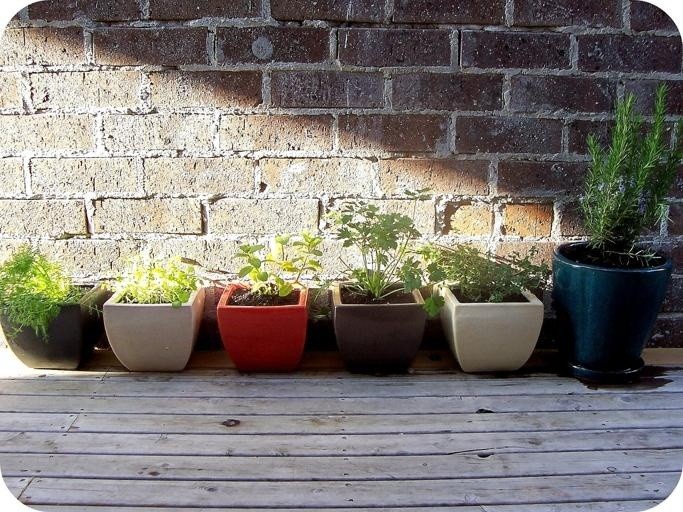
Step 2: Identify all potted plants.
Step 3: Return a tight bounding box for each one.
[0,240,106,370]
[405,227,552,372]
[216,231,323,370]
[102,253,205,370]
[321,185,444,375]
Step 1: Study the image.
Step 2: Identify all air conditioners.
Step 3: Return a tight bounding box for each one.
[550,82,674,385]
